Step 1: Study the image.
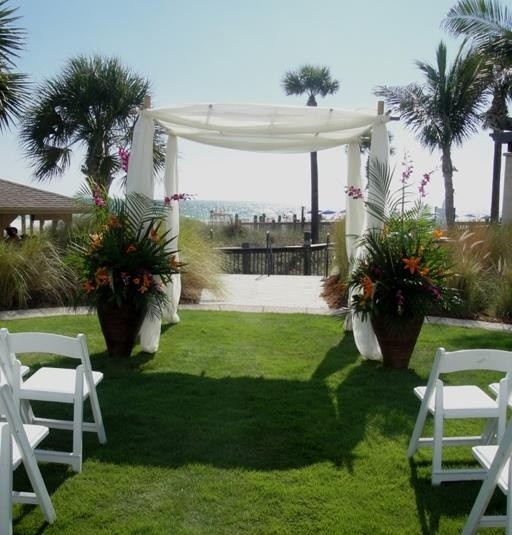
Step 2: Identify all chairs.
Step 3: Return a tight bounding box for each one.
[406,347,512,535]
[0,328,108,534]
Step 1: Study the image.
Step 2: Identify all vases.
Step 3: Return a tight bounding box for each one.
[371,314,424,369]
[97,304,146,357]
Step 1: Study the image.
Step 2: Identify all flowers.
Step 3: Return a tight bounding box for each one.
[321,147,465,343]
[48,145,192,324]
